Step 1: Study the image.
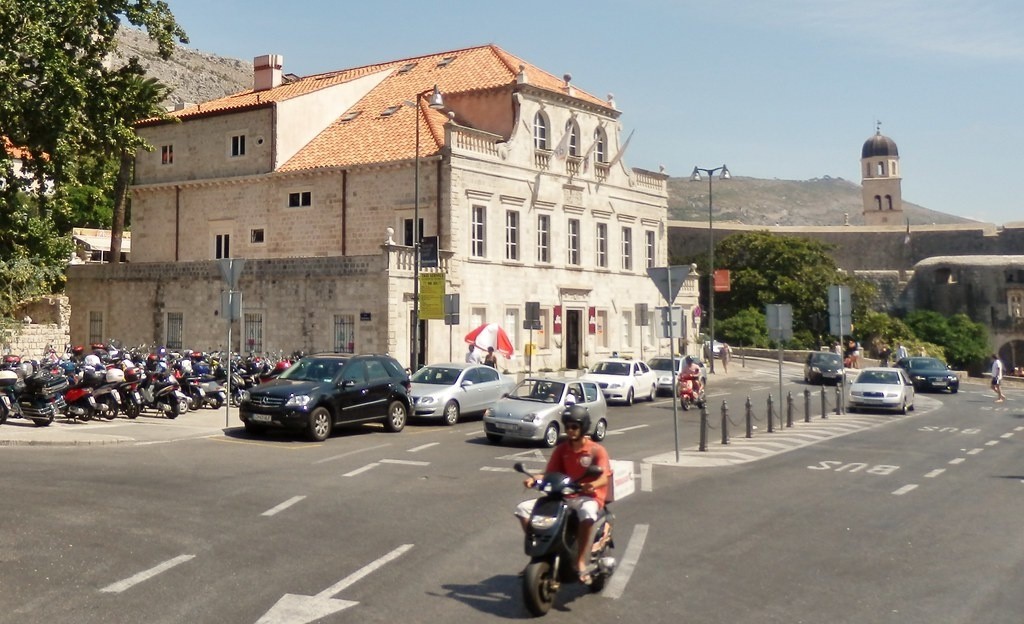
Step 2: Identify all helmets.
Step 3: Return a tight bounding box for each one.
[685,355,693,363]
[561,405,590,436]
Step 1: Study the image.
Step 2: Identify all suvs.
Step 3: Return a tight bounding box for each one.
[239,352,415,443]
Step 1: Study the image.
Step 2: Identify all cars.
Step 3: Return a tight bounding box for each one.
[646,354,706,398]
[483,377,609,449]
[804,352,846,385]
[578,358,657,407]
[699,333,733,360]
[848,367,915,415]
[409,362,516,426]
[892,356,959,394]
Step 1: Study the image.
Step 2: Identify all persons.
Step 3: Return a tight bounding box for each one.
[896,341,908,361]
[523,404,612,585]
[612,351,618,357]
[829,340,840,354]
[719,342,731,375]
[678,355,703,401]
[842,339,860,368]
[990,353,1006,403]
[484,346,498,369]
[1009,367,1024,376]
[466,343,483,364]
[549,386,575,403]
[878,341,893,367]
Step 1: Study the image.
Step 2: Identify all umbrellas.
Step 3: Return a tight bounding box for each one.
[465,323,514,359]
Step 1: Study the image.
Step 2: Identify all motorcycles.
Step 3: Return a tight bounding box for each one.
[674,368,707,411]
[0,338,305,427]
[513,462,617,616]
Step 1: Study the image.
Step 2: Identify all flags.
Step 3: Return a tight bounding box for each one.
[555,127,572,163]
[610,135,631,167]
[587,129,605,160]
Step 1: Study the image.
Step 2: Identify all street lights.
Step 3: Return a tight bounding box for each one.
[689,164,732,374]
[412,85,445,372]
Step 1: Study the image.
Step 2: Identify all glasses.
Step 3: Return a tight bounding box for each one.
[564,423,582,432]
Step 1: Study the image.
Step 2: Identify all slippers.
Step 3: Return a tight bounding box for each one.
[576,566,592,584]
